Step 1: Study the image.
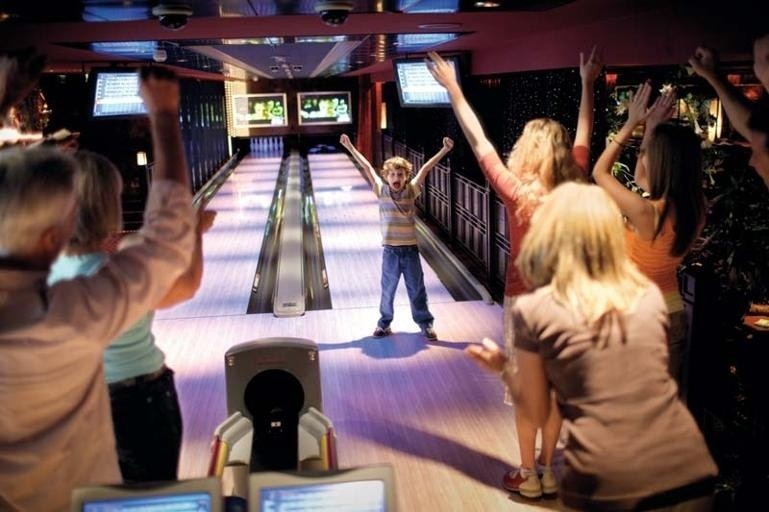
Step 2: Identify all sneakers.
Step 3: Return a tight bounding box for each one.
[372,326,392,338]
[534,462,558,494]
[501,466,542,498]
[421,325,437,341]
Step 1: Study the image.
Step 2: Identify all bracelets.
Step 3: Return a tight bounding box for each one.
[499,365,513,379]
[608,132,628,148]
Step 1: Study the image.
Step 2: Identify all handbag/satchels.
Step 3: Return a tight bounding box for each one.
[692,249,752,336]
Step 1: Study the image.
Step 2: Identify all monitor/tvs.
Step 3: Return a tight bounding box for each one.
[80,6,158,59]
[391,55,462,109]
[232,92,289,129]
[296,91,354,127]
[396,0,459,54]
[90,71,154,118]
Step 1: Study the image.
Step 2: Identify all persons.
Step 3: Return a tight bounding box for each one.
[688,37,769,194]
[47,151,216,485]
[340,132,454,342]
[424,45,605,501]
[462,180,718,512]
[591,81,709,398]
[0,62,197,512]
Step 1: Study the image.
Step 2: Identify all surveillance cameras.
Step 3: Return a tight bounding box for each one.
[315,3,354,27]
[151,6,194,31]
